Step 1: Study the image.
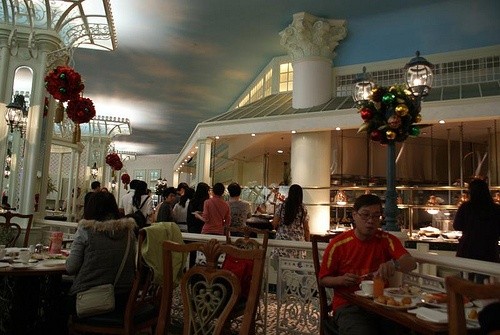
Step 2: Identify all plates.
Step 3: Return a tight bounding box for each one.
[465,308,484,322]
[374,297,417,308]
[383,287,422,297]
[355,290,388,298]
[0,247,70,268]
[192,212,203,215]
[421,293,473,308]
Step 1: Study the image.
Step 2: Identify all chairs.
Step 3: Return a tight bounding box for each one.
[0,204,500,335]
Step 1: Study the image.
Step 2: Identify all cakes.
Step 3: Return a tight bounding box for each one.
[423,292,448,303]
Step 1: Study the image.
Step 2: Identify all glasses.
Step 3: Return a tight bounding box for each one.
[356,211,380,222]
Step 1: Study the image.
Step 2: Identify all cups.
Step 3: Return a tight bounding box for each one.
[0,245,5,257]
[20,249,30,264]
[359,281,374,295]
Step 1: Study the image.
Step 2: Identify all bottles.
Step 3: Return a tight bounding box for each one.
[35,243,43,254]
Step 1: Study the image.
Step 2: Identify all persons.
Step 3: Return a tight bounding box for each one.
[271,184,310,259]
[453,179,500,284]
[318,194,416,335]
[62,180,252,237]
[41,192,137,335]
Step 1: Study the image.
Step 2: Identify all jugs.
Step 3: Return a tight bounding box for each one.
[48,232,63,255]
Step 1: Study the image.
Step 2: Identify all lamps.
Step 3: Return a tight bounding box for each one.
[91,162,98,179]
[112,180,115,190]
[4,149,12,178]
[4,95,29,138]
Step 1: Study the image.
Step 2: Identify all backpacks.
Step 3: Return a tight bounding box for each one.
[132,195,151,227]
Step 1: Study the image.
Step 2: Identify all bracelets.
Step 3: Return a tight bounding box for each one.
[394,261,400,271]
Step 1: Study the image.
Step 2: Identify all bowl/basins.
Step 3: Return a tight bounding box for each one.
[402,227,462,239]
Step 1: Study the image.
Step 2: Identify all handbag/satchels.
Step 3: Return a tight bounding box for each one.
[77,284,115,317]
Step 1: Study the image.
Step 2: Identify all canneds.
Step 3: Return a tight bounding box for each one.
[35,243,43,255]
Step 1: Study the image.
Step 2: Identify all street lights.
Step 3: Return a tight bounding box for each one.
[352,50,434,231]
[157,176,168,202]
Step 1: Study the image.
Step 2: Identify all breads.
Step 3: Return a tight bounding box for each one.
[377,294,411,307]
[467,308,479,319]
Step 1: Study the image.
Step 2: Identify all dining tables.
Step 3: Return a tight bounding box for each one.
[0,248,67,318]
[351,293,450,335]
